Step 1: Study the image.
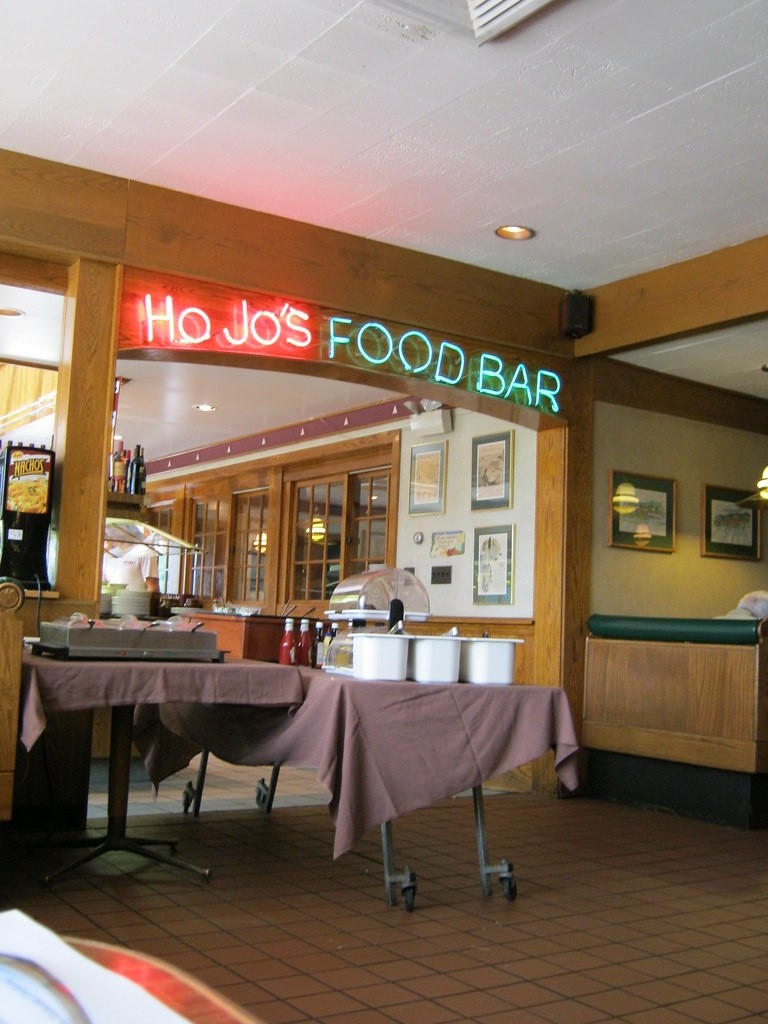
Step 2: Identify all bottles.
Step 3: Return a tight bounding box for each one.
[329,624,339,647]
[297,619,312,666]
[313,622,325,668]
[280,618,296,666]
[112,440,147,495]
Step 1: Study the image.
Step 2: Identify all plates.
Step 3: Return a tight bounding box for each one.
[100,590,152,619]
[171,607,200,615]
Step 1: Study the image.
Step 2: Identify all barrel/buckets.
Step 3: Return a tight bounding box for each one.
[408,635,469,683]
[461,636,525,684]
[347,634,414,681]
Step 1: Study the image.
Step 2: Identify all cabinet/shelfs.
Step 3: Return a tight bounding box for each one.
[180,611,331,662]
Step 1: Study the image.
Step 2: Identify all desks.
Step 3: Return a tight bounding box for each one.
[294,664,581,911]
[16,652,305,892]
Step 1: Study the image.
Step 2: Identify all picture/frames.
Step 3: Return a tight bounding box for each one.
[607,468,678,553]
[471,429,514,511]
[471,523,516,605]
[700,480,762,562]
[407,440,449,517]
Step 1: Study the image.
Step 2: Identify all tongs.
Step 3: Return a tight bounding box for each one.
[387,620,404,635]
[441,626,460,637]
